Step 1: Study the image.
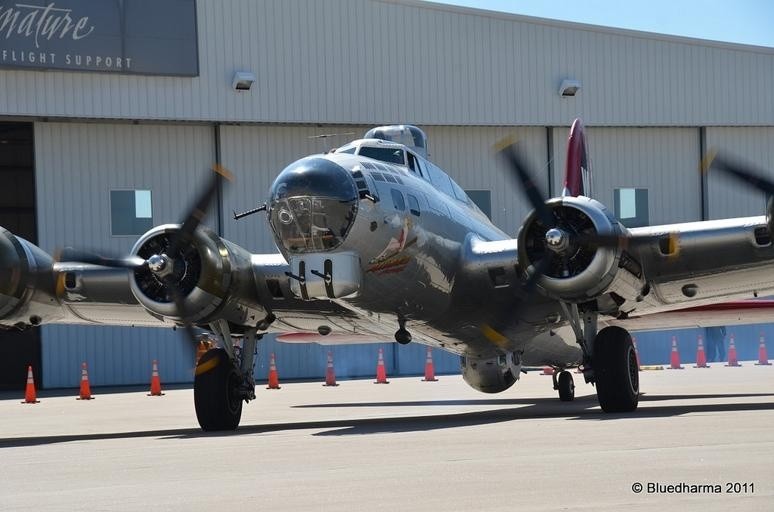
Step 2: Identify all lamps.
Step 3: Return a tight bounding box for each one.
[558,78,581,101]
[231,69,254,91]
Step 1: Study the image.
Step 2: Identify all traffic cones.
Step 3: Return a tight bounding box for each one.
[631,335,642,371]
[539,368,554,375]
[265,352,282,389]
[692,335,710,368]
[75,360,94,400]
[724,333,741,366]
[665,335,684,369]
[574,366,583,373]
[146,359,164,396]
[20,366,40,403]
[322,350,339,386]
[754,330,772,365]
[373,347,390,384]
[421,346,437,381]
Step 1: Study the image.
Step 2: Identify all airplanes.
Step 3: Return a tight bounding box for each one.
[0,123,773,432]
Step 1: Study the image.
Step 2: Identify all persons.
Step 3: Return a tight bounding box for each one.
[754,290,759,298]
